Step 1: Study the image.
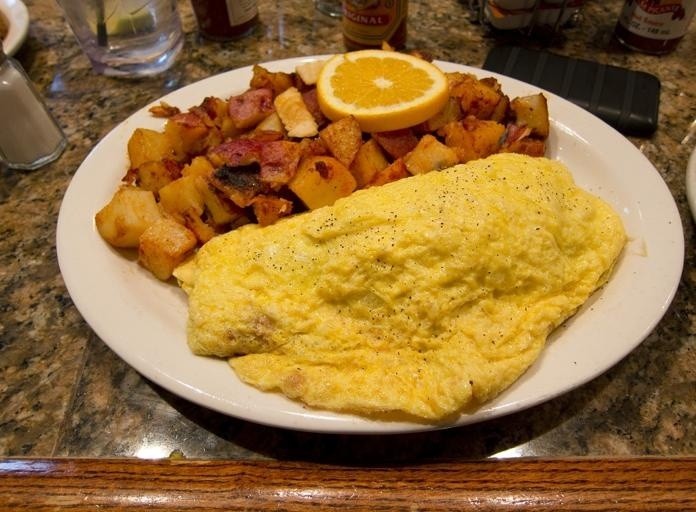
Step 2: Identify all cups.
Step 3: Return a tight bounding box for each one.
[55,1,186,79]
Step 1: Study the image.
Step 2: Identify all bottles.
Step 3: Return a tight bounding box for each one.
[614,1,695,56]
[190,2,262,42]
[0,39,68,171]
[343,0,407,52]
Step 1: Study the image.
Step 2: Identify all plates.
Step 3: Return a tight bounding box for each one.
[56,52,685,435]
[0,1,30,57]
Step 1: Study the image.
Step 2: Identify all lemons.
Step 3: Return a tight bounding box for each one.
[317,48,447,133]
